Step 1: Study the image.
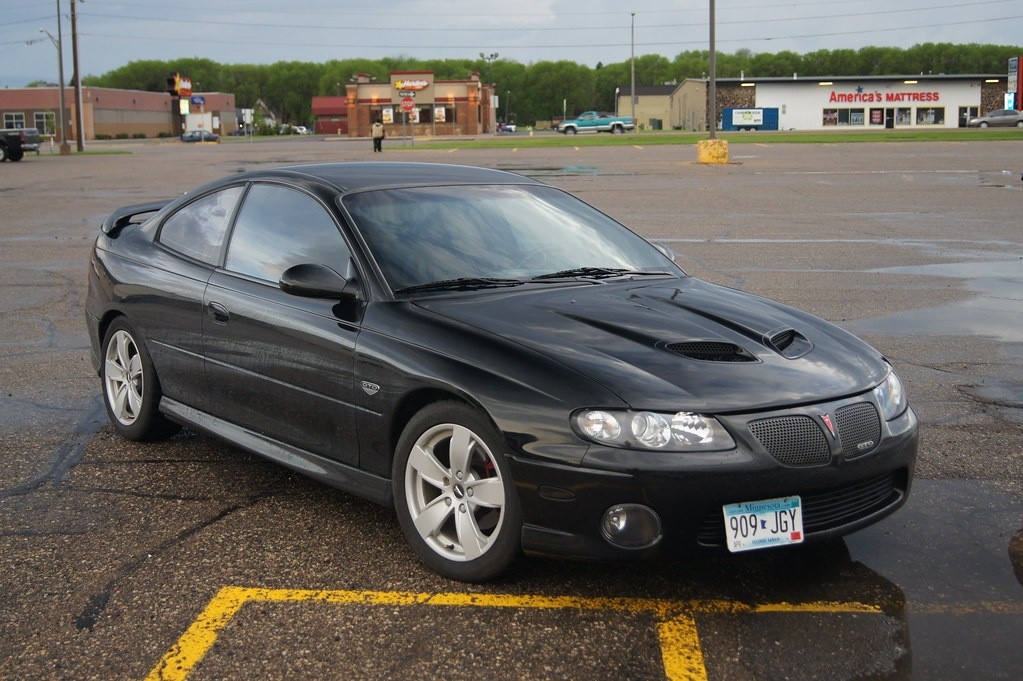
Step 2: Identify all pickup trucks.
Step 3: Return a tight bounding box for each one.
[496,122,517,133]
[557,110,637,136]
[0,127,44,163]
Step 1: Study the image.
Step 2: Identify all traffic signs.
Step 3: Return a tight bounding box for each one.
[399,91,417,98]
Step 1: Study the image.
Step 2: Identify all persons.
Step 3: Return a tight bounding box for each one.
[499,121,503,133]
[526,122,531,136]
[371,119,385,153]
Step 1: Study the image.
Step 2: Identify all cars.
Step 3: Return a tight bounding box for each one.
[967,110,1023,129]
[181,128,220,143]
[292,125,308,135]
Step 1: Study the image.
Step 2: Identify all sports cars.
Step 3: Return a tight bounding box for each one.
[81,161,919,587]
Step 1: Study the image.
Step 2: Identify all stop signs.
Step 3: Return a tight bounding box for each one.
[400,96,416,112]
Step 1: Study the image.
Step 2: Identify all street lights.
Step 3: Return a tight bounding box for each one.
[506,90,510,126]
[195,81,204,143]
[68,0,85,151]
[37,28,67,144]
[629,12,637,118]
[614,87,621,135]
[479,51,499,84]
